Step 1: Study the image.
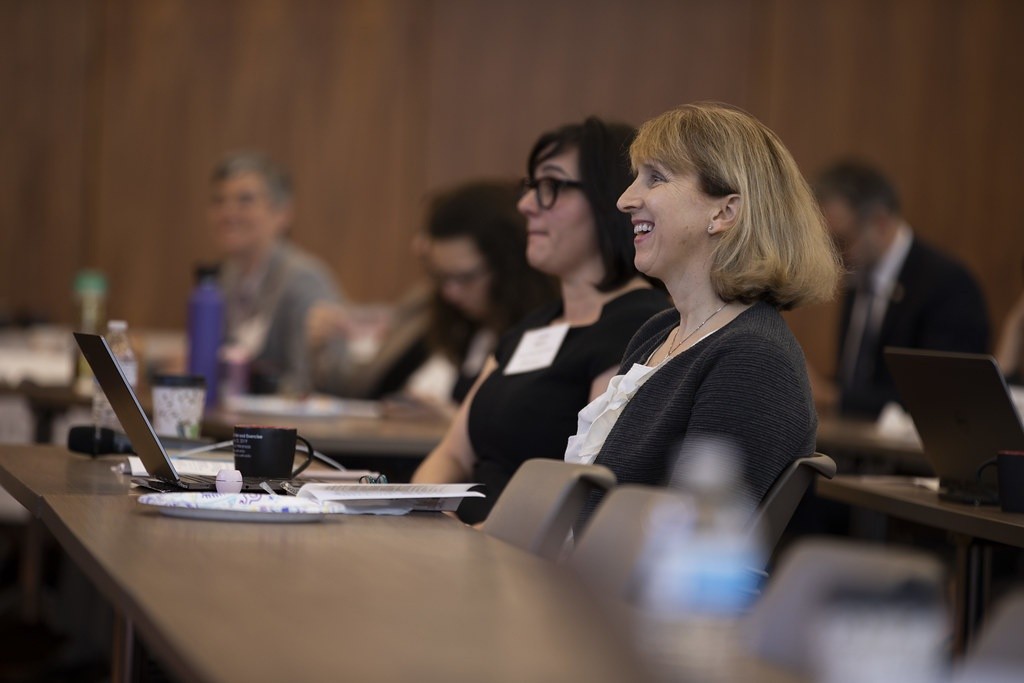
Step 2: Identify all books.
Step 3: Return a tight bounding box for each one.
[292,483,485,511]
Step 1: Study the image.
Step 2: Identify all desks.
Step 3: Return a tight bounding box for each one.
[0,329,1024,683]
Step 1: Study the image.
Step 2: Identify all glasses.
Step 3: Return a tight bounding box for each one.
[418,261,488,288]
[518,175,581,211]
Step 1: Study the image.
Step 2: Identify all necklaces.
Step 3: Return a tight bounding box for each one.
[665,304,728,359]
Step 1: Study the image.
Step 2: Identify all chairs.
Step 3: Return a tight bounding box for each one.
[707,451,838,628]
[485,457,617,556]
[334,279,447,400]
[554,484,718,608]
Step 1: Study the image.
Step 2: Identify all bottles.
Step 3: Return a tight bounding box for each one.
[91,320,139,442]
[185,260,225,403]
[637,435,763,683]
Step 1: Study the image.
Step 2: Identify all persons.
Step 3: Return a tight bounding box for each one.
[307,171,563,483]
[560,105,843,559]
[804,158,1024,651]
[208,149,345,397]
[410,115,675,529]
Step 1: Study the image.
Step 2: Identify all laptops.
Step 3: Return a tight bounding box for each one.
[72,331,366,492]
[883,346,1024,501]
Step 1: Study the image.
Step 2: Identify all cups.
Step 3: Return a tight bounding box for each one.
[797,543,951,683]
[343,302,395,364]
[232,425,314,477]
[69,266,109,382]
[151,373,208,441]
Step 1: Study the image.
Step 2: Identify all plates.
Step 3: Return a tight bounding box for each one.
[138,491,346,523]
